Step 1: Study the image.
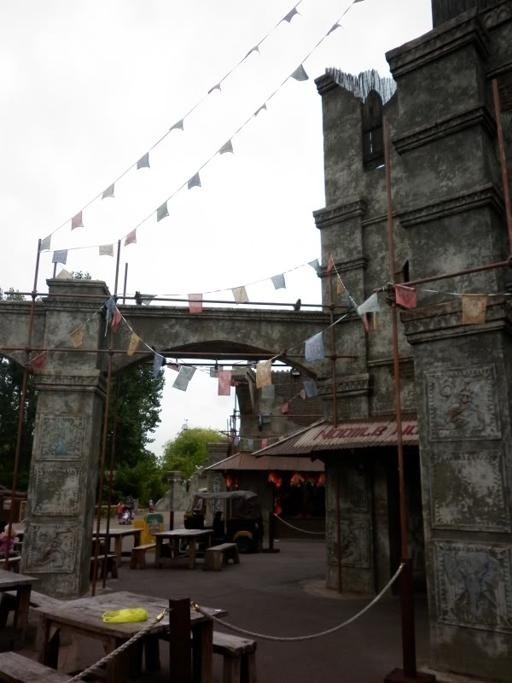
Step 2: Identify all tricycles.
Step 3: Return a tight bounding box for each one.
[178,490,265,555]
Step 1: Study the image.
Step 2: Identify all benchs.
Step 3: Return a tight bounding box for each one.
[1,542,258,683]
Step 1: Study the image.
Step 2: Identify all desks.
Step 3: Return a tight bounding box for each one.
[152,528,213,569]
[91,528,143,567]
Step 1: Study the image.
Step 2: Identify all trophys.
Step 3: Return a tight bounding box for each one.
[99,243,116,256]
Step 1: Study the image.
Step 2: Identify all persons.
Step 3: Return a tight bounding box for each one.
[115,497,122,519]
[146,498,157,514]
[123,493,135,520]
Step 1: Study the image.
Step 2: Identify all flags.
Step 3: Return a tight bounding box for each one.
[232,286,249,303]
[105,297,122,333]
[168,362,179,370]
[137,6,299,169]
[52,249,72,264]
[271,274,286,290]
[462,294,486,325]
[210,366,223,378]
[188,293,202,314]
[153,353,164,377]
[327,259,334,272]
[156,24,344,222]
[232,437,243,448]
[309,259,323,276]
[356,292,380,313]
[137,292,154,305]
[260,438,268,451]
[254,360,271,389]
[124,228,139,246]
[127,332,141,356]
[305,331,325,361]
[280,380,317,414]
[29,350,48,367]
[172,365,197,392]
[218,370,231,395]
[101,183,115,199]
[247,438,255,453]
[38,234,53,253]
[70,212,86,230]
[394,283,416,308]
